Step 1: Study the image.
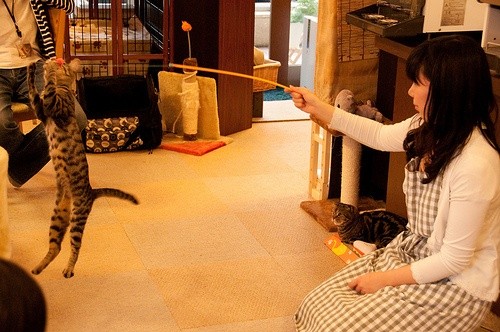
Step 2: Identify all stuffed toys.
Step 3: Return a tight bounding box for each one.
[335,89,383,123]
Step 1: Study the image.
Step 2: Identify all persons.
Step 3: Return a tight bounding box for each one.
[283,34,499,332]
[1,0,75,189]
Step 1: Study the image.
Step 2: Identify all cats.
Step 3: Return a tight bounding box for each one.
[26,56,140,279]
[332,199,408,249]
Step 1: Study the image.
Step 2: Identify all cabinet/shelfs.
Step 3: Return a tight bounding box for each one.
[374,35,500,222]
